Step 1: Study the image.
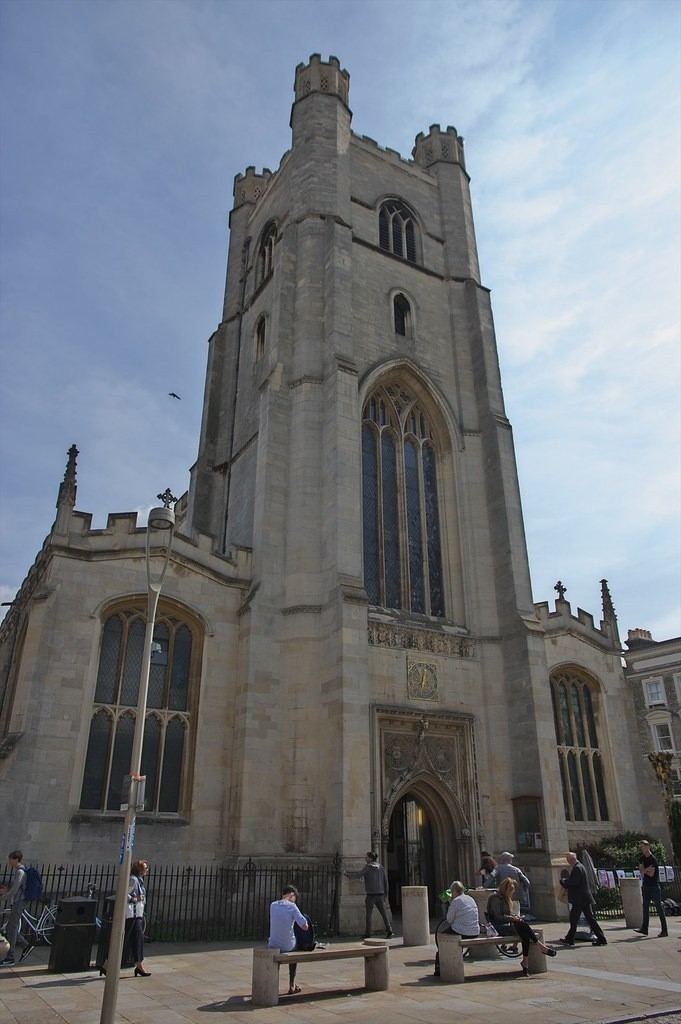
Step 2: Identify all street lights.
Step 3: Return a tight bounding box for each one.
[98,504,178,1024]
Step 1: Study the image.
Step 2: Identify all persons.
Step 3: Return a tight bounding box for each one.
[483,852,531,953]
[484,878,557,977]
[435,881,480,949]
[0,850,35,967]
[480,858,496,878]
[559,852,608,946]
[268,885,308,993]
[633,840,669,937]
[344,851,394,939]
[100,860,153,976]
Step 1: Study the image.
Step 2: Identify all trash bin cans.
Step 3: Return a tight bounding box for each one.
[48,895,99,972]
[96,893,135,969]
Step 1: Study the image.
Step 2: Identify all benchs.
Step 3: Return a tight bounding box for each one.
[253,939,389,1006]
[437,927,547,984]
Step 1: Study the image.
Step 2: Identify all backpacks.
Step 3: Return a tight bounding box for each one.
[294,914,317,951]
[15,866,43,900]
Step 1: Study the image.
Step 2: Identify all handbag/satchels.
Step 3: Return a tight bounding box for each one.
[557,885,568,904]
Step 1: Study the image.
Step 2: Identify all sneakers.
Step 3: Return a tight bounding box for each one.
[0,957,15,966]
[19,945,35,963]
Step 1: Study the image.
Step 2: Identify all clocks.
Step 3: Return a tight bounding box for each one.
[407,660,440,702]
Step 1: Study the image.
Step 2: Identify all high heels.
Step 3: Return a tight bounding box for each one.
[134,967,151,977]
[100,967,106,976]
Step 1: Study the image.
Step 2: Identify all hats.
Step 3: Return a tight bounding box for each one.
[501,851,514,860]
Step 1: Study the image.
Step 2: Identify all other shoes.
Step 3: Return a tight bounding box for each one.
[386,931,394,939]
[634,929,648,935]
[361,936,368,941]
[520,962,529,977]
[560,938,574,945]
[289,986,301,994]
[542,949,556,956]
[592,941,607,946]
[657,932,668,937]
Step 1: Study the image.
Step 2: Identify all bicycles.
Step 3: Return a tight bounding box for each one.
[435,918,525,958]
[0,896,58,945]
